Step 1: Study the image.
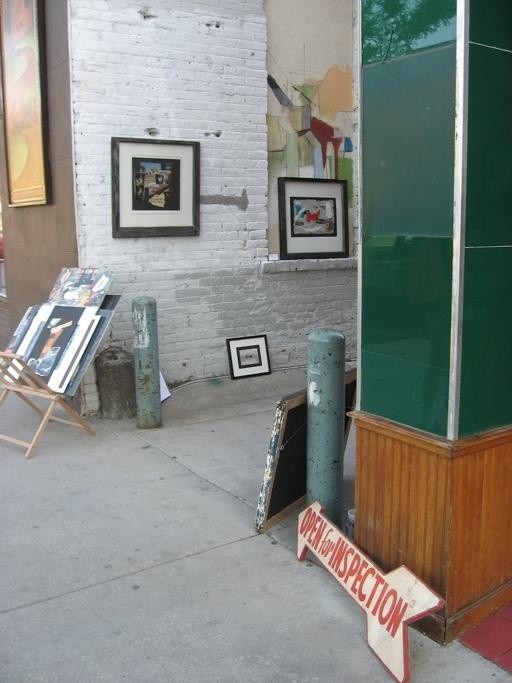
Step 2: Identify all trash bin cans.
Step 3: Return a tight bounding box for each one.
[94,346,137,420]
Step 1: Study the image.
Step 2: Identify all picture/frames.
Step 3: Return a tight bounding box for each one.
[277,175,349,259]
[224,333,272,380]
[110,135,200,237]
[254,367,357,535]
